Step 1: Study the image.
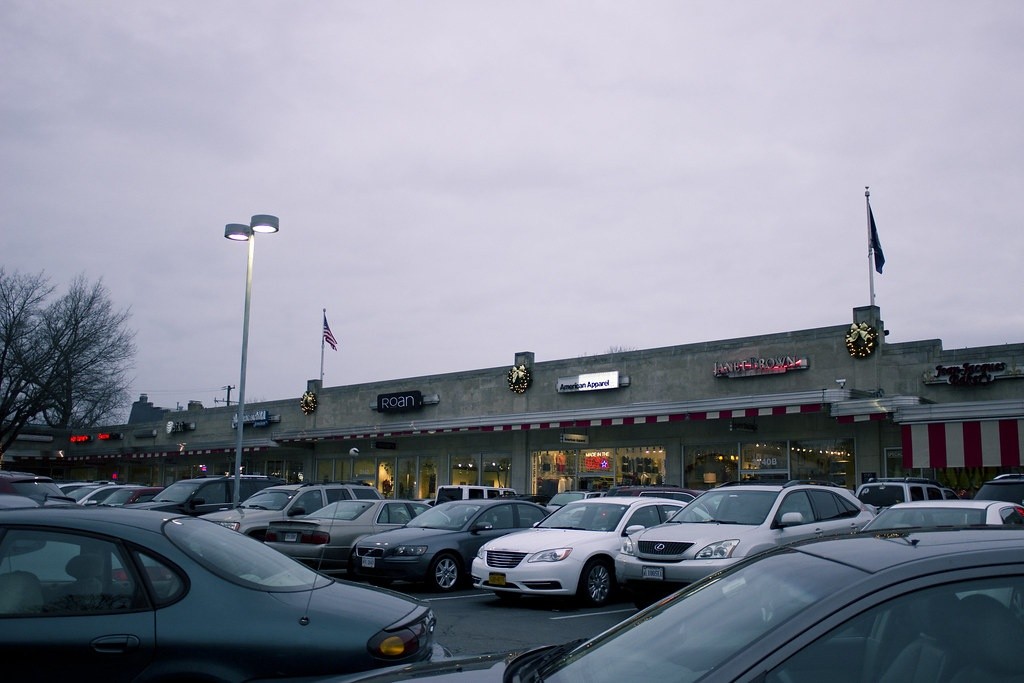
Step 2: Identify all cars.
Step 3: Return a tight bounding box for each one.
[263,498,452,573]
[0,471,164,509]
[472,496,714,609]
[546,488,718,522]
[0,507,436,683]
[858,501,1024,611]
[316,524,1024,683]
[352,499,554,592]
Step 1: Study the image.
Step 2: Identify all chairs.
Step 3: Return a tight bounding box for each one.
[65,553,104,612]
[0,570,43,616]
[861,602,907,683]
[715,496,750,524]
[877,627,957,683]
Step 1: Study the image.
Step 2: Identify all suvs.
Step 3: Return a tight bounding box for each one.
[615,478,876,607]
[122,473,288,517]
[197,481,383,544]
[435,485,517,507]
[855,474,1024,515]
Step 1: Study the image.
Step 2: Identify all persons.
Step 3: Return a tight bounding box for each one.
[957,488,971,499]
[541,450,573,492]
[622,453,660,473]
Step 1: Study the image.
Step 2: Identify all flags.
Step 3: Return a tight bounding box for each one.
[869,206,885,274]
[323,315,337,350]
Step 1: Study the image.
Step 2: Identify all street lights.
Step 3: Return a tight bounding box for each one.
[224,214,279,510]
